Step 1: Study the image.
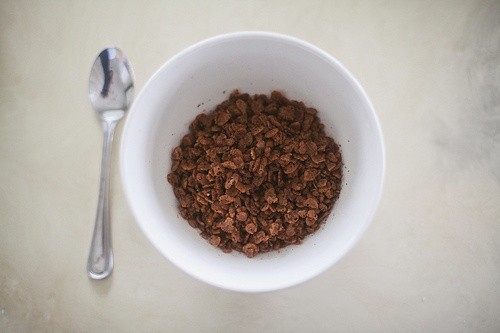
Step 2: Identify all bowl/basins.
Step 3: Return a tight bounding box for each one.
[117,30,386,294]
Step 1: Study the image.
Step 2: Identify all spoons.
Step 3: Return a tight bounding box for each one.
[86,47,137,279]
